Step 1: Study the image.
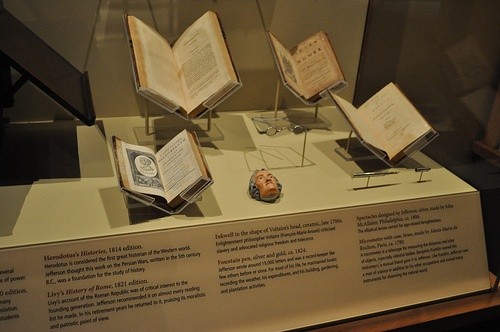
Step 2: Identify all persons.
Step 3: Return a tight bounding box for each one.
[249,168,282,201]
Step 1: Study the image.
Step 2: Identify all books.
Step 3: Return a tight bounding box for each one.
[266,29,349,106]
[122,11,243,121]
[109,128,214,215]
[327,82,440,168]
